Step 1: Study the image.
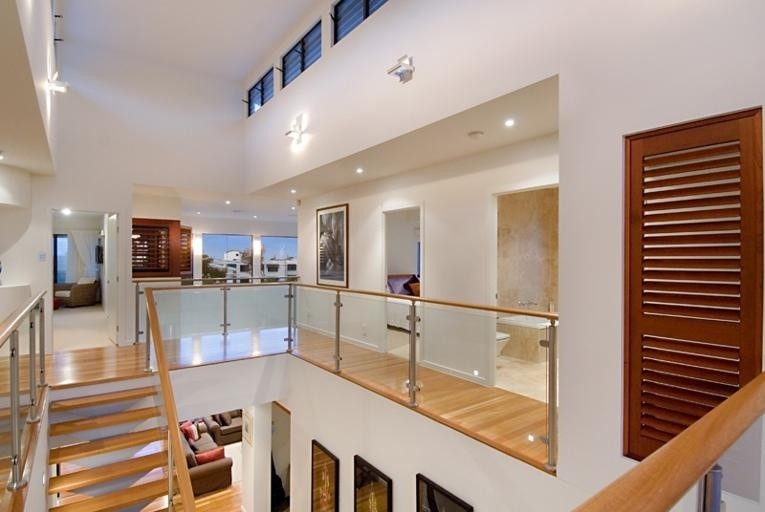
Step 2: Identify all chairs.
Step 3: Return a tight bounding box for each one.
[54,276,100,307]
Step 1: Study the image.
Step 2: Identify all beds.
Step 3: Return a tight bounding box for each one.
[386,274,420,334]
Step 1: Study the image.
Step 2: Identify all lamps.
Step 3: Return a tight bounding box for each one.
[46,69,70,92]
[383,53,414,86]
[284,114,305,143]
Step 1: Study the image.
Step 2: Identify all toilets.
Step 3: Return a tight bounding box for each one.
[496,330,510,356]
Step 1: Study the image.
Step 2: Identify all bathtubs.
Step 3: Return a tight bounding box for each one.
[496,314,559,363]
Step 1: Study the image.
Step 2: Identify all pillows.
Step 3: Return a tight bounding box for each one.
[213,412,232,426]
[195,446,226,465]
[388,275,420,297]
[76,276,96,285]
[180,420,210,450]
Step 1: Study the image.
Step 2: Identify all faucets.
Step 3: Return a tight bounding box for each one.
[518,300,539,308]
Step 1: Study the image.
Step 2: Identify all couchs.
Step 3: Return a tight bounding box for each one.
[202,409,242,446]
[179,421,233,496]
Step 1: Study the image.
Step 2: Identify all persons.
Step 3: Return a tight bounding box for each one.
[320,226,343,270]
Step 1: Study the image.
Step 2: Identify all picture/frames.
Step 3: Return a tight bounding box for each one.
[311,438,339,510]
[415,474,475,511]
[315,204,348,288]
[354,454,391,511]
